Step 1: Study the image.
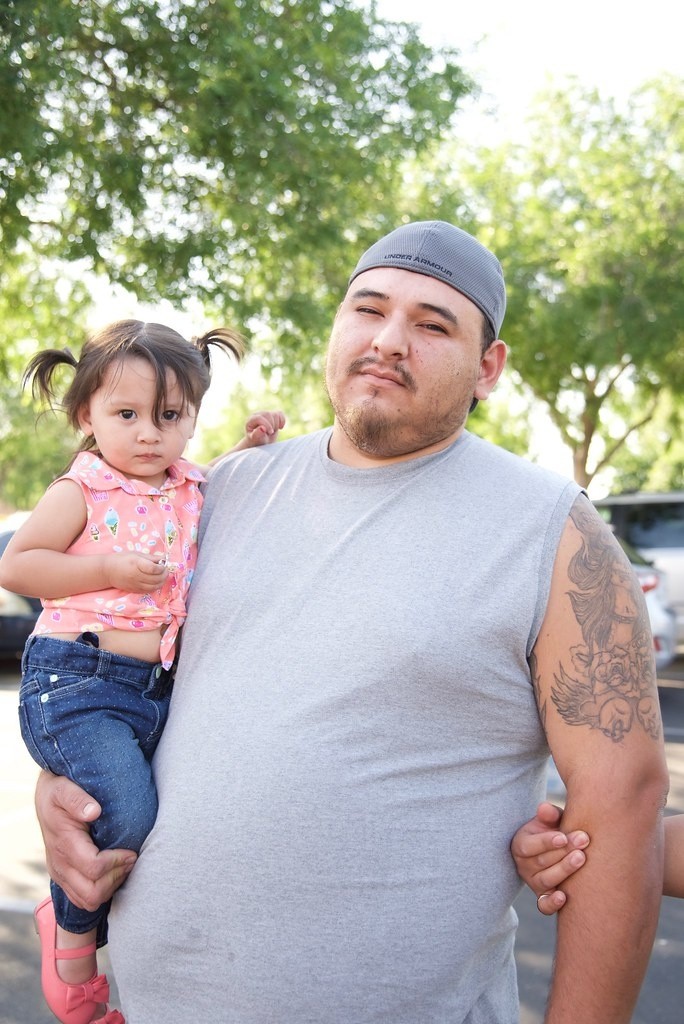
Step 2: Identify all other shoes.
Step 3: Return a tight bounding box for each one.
[89,1002,125,1024]
[34,895,109,1024]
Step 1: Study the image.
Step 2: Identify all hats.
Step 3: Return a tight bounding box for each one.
[349,220,507,340]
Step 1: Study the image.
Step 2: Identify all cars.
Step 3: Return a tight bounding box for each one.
[630,563,680,670]
[0,510,44,672]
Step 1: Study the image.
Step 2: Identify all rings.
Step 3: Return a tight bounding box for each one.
[536,894,556,916]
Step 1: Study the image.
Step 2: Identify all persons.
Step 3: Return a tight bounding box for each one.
[510,801,684,916]
[0,317,286,1024]
[33,220,669,1023]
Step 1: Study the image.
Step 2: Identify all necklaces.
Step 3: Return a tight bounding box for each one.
[122,472,180,572]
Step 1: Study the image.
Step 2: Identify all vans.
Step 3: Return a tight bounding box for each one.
[585,491,684,646]
